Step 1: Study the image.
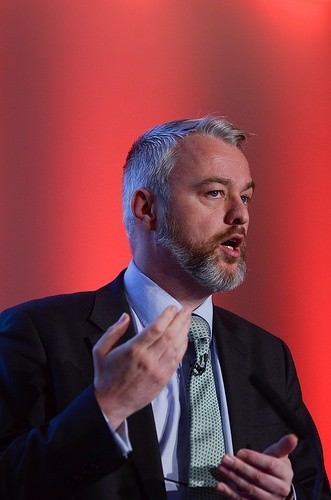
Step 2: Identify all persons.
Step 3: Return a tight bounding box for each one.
[0,115,331,500]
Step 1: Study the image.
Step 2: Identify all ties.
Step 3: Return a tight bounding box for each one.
[188,315,226,488]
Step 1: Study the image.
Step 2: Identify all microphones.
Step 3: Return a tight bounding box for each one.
[195,353,208,375]
